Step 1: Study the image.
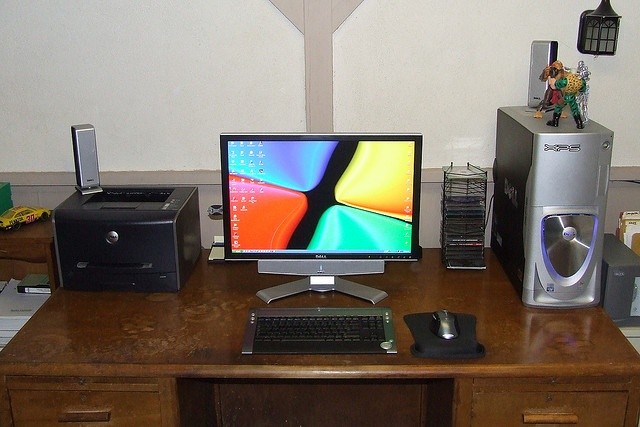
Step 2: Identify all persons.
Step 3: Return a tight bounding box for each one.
[546,66,586,129]
[573,60,591,123]
[533,60,571,119]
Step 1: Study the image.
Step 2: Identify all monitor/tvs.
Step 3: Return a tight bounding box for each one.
[219,132,423,305]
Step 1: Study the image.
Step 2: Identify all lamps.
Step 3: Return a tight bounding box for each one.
[577,0,622,55]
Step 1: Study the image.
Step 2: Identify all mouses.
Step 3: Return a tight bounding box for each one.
[429,308,461,340]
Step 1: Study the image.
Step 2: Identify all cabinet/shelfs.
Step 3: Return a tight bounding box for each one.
[439,163,489,270]
[0,208,61,295]
[0,248,640,427]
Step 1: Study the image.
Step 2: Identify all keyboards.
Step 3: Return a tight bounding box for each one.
[241,307,398,355]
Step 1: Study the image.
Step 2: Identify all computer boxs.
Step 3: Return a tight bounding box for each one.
[490,107,614,311]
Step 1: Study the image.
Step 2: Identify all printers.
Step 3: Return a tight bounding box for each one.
[50,185,202,293]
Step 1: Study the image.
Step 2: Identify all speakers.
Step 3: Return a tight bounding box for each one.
[525,40,558,114]
[600,231,640,328]
[71,124,104,195]
